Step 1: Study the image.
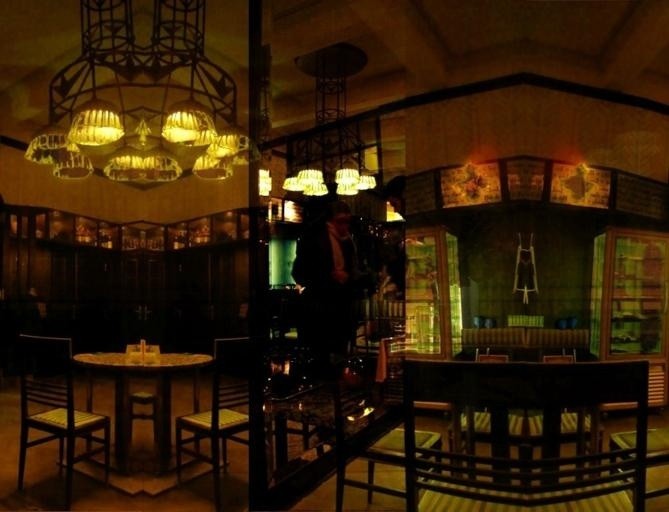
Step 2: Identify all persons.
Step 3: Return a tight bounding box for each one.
[386,176,402,216]
[291,202,376,369]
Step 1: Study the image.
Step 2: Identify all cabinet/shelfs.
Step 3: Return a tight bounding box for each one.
[406,224,463,358]
[586,224,668,420]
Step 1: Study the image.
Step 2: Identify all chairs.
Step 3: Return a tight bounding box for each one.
[17,334,112,512]
[610,426,669,511]
[402,359,649,511]
[446,327,604,487]
[327,347,442,511]
[175,336,249,512]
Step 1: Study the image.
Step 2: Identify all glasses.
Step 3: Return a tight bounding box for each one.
[335,217,353,225]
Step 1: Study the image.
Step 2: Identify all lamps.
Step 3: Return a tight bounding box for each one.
[23,0,261,184]
[280,39,378,197]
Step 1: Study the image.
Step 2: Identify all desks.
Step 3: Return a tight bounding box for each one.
[72,352,213,474]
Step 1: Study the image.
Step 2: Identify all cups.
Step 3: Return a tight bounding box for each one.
[125,345,142,364]
[143,344,161,363]
